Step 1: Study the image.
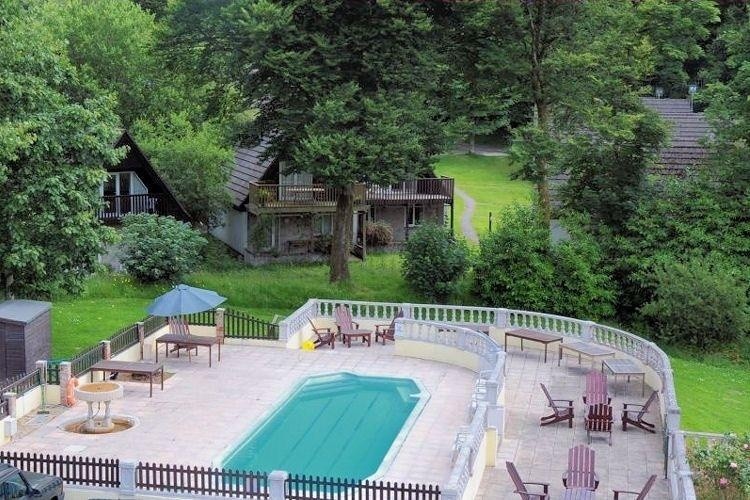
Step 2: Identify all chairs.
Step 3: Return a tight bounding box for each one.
[507,448,654,499]
[540,369,658,446]
[307,306,402,350]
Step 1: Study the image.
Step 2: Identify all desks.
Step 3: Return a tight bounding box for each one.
[438,322,646,398]
[91,361,163,397]
[155,334,220,368]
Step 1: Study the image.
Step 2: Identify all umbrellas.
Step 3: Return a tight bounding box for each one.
[143,284,229,335]
